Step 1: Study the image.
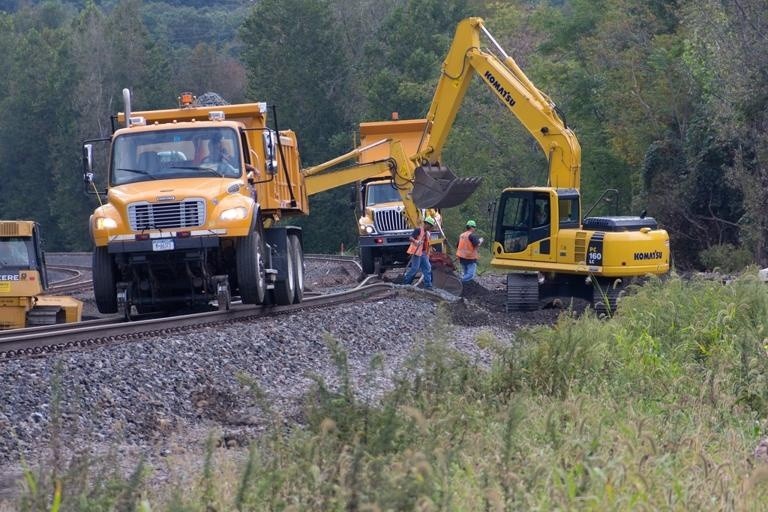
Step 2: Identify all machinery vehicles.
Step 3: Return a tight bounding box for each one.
[409,16,671,318]
[0,217,83,329]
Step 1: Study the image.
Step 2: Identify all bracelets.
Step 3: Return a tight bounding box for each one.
[415,240,417,242]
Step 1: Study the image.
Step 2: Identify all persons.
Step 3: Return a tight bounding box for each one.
[456,220,483,281]
[208,139,260,177]
[404,217,435,291]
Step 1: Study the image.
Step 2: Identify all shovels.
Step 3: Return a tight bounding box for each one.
[394,232,427,285]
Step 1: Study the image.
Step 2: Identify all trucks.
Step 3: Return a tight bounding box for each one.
[355,111,445,274]
[78,88,306,321]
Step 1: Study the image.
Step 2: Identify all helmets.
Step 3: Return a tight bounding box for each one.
[424,216,435,226]
[467,220,476,226]
